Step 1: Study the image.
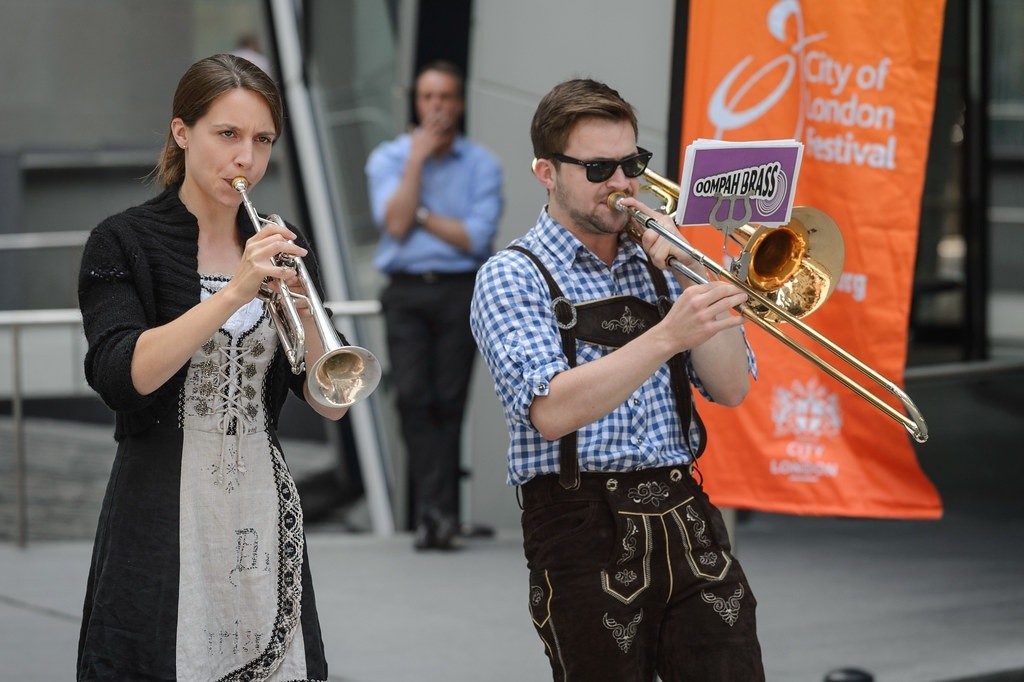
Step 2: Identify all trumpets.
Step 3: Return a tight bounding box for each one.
[231,175,382,410]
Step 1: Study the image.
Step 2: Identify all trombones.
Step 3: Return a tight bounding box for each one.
[605,163,930,443]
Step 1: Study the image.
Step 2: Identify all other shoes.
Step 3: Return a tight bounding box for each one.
[430,513,456,547]
[414,525,432,548]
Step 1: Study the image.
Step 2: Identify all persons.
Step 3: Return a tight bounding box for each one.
[77,53,352,682]
[231,33,269,73]
[473,80,766,682]
[364,61,504,535]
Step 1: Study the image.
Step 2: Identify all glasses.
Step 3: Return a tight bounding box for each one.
[547,146,653,182]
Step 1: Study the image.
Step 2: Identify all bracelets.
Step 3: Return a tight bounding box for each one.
[416,206,429,225]
